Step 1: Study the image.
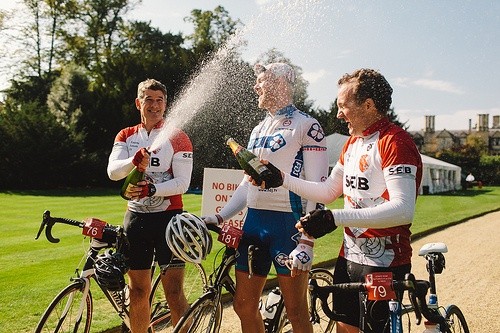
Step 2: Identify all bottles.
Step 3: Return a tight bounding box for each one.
[120,151,151,201]
[422,320,440,333]
[263,287,282,320]
[117,283,130,312]
[224,135,276,189]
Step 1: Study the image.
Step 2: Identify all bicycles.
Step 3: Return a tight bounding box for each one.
[172,210,340,333]
[309,242,471,333]
[33,210,208,333]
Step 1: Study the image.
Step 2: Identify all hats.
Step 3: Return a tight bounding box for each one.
[254,62,296,87]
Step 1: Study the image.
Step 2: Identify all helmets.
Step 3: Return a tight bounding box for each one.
[92,252,126,291]
[164,212,213,265]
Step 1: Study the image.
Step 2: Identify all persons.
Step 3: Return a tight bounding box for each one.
[201,62,328,333]
[107,78,193,333]
[244,66,423,333]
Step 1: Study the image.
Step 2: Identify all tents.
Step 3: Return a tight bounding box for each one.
[323,133,461,195]
